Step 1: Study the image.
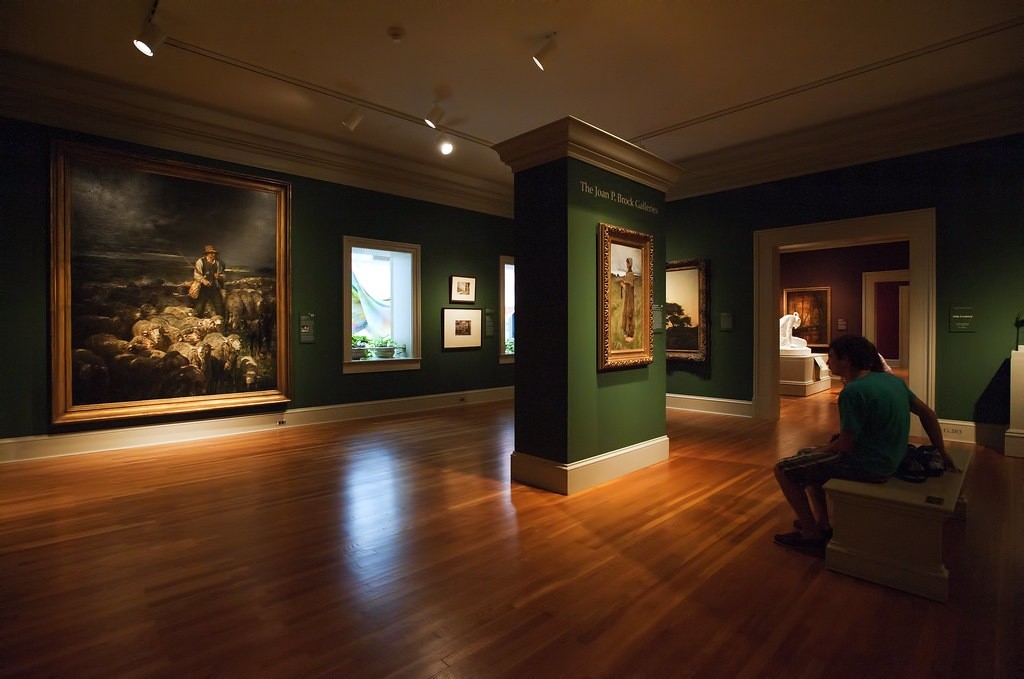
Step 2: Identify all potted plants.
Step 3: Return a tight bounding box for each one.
[505,338,515,354]
[371,337,401,358]
[352,336,370,358]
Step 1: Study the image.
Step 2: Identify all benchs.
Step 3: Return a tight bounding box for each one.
[820,434,976,604]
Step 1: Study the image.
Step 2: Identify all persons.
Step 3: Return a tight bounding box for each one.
[772,336,956,553]
[797,337,894,454]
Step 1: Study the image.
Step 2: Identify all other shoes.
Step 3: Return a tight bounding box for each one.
[794,520,833,541]
[774,532,827,552]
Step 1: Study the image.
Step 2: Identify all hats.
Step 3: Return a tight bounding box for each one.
[204,246,219,253]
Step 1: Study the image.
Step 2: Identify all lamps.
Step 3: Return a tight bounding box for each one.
[425,94,445,129]
[533,32,560,71]
[341,102,364,131]
[133,14,167,57]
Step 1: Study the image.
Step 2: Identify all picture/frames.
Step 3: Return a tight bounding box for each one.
[594,221,654,374]
[783,286,832,347]
[450,275,477,303]
[50,138,294,426]
[441,308,484,351]
[665,257,711,363]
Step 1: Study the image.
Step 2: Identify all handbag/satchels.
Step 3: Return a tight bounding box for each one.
[894,444,946,483]
[189,275,206,298]
